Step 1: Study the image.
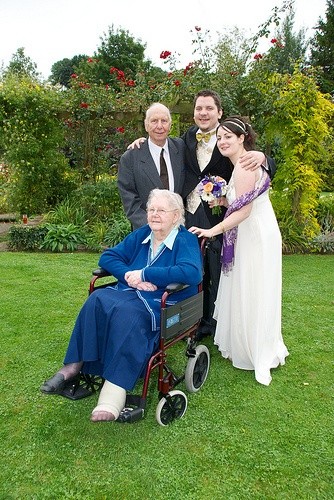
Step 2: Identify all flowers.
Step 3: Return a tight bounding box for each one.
[196,172,227,217]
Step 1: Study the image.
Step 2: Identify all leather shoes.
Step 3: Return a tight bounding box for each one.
[192,326,211,342]
[39,366,81,395]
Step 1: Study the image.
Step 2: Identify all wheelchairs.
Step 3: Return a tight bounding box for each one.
[55,235,214,427]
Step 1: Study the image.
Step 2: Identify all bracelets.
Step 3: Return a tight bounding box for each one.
[220,222,225,233]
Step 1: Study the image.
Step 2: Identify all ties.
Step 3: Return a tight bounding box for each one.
[160,148,169,192]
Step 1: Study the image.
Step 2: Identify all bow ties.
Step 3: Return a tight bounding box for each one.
[196,130,217,143]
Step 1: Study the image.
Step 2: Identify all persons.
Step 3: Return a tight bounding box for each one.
[40,189,203,422]
[120,90,276,341]
[187,115,289,386]
[118,103,187,234]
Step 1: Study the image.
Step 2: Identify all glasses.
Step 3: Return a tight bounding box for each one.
[146,206,178,215]
